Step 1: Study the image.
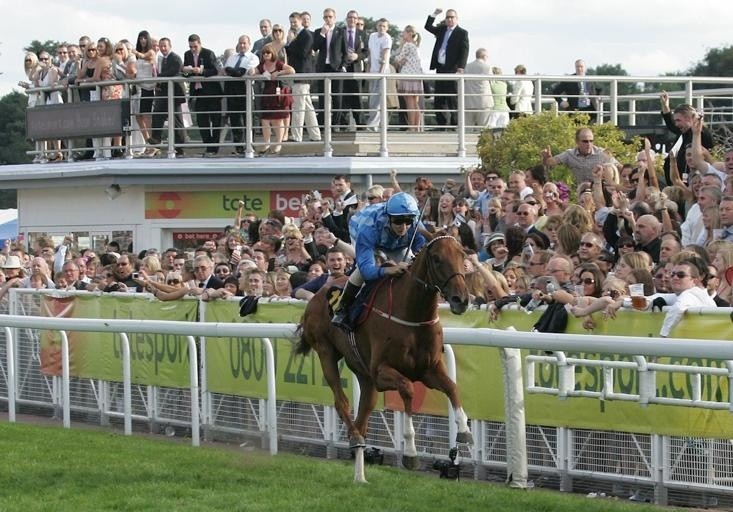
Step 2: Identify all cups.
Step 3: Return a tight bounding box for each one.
[574,285,584,298]
[628,283,645,308]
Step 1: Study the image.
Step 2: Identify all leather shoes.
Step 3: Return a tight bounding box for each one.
[33,147,273,163]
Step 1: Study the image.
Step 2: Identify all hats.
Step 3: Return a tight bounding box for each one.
[0,256,21,269]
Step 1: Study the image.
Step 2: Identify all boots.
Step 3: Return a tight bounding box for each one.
[331,280,361,332]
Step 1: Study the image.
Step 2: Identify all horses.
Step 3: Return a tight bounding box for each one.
[286,222,472,488]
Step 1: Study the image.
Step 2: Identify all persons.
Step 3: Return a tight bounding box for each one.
[414,89,733,338]
[24,7,534,158]
[552,59,602,126]
[0,169,403,302]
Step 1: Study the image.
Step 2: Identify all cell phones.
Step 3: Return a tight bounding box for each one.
[336,201,346,210]
[522,242,534,259]
[692,113,703,122]
[132,273,139,279]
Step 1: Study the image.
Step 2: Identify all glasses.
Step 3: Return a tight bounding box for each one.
[25,28,284,61]
[324,15,454,25]
[390,218,414,225]
[117,195,376,285]
[452,139,719,284]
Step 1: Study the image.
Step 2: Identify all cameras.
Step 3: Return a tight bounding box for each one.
[351,447,385,465]
[18,232,25,240]
[433,447,466,482]
[545,192,553,198]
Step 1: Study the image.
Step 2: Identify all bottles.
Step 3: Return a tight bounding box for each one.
[524,293,543,315]
[545,280,555,295]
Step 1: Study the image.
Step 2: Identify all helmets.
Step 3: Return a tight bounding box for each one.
[387,193,420,217]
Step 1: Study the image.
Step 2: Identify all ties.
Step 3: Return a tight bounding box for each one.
[348,30,354,49]
[439,29,451,57]
